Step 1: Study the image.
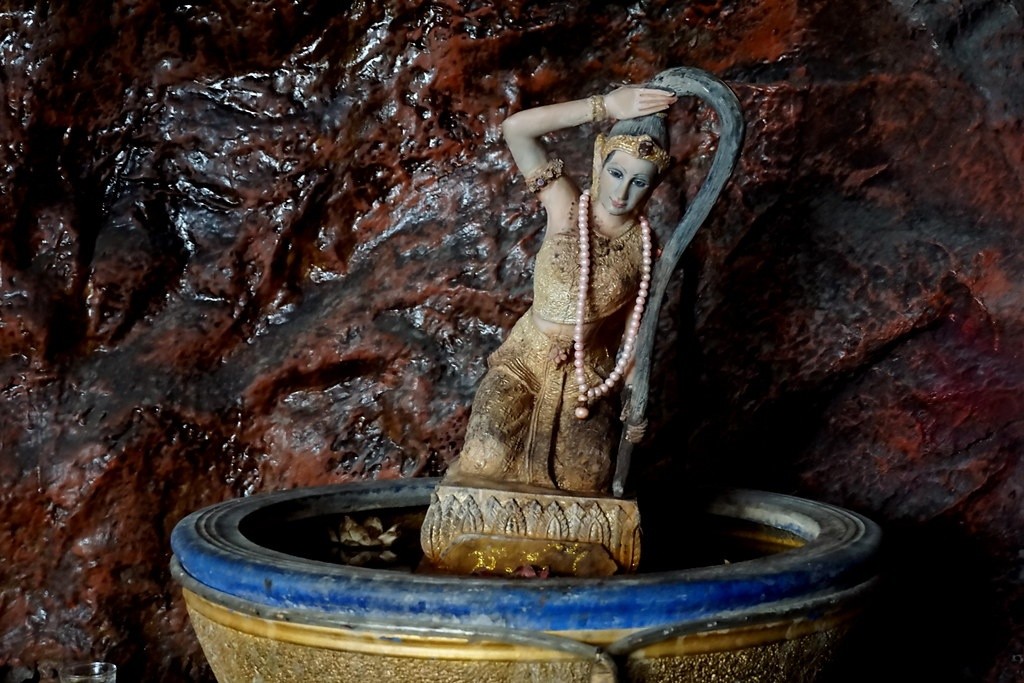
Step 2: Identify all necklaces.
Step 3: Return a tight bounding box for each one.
[575,187,652,420]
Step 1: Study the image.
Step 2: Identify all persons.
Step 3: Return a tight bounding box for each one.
[460,65,745,497]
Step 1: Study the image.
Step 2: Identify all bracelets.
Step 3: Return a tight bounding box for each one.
[591,93,608,130]
